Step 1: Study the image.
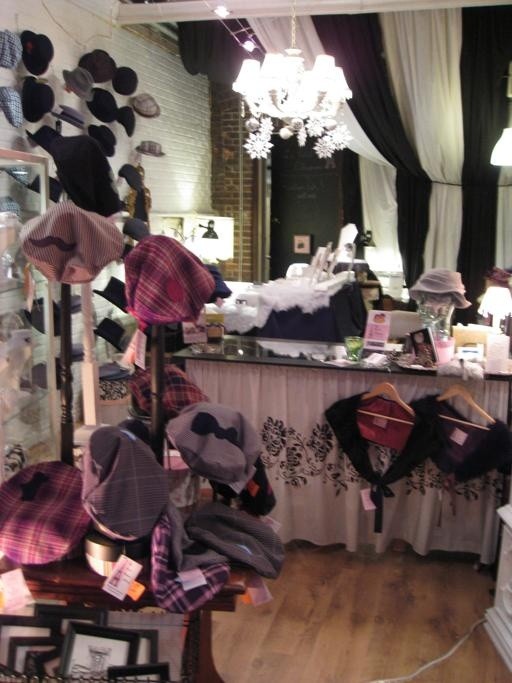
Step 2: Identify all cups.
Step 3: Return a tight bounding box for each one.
[343,335,365,359]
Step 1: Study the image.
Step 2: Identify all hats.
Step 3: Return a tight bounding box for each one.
[408,267,473,309]
[122,234,233,330]
[0,366,286,615]
[0,27,165,393]
[484,267,512,288]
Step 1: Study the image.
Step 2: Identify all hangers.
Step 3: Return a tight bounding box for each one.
[326,366,511,438]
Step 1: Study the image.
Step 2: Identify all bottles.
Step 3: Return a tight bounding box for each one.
[205,313,225,346]
